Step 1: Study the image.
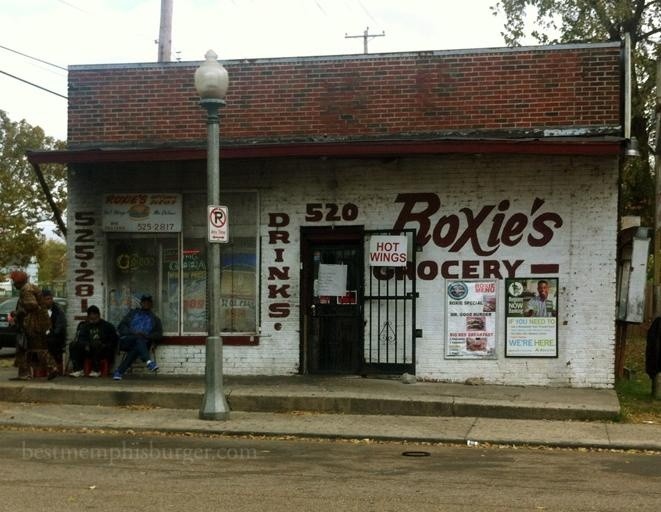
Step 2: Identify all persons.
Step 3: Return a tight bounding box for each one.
[40,289,68,377]
[113,294,162,380]
[524,280,554,317]
[9,270,52,381]
[67,305,118,378]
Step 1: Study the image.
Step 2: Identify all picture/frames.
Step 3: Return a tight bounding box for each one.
[505,277,558,358]
[445,278,498,360]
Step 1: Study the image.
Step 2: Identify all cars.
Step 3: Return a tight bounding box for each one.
[1,296,68,351]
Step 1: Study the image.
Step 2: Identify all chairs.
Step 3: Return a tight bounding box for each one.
[63,322,157,379]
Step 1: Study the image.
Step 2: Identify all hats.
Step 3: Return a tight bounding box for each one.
[141,293,153,302]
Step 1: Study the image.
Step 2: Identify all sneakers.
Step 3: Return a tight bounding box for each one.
[48,365,60,381]
[68,368,88,378]
[113,368,122,380]
[89,371,102,377]
[146,361,158,372]
[8,374,34,380]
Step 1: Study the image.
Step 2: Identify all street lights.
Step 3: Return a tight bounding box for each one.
[195,49,232,421]
